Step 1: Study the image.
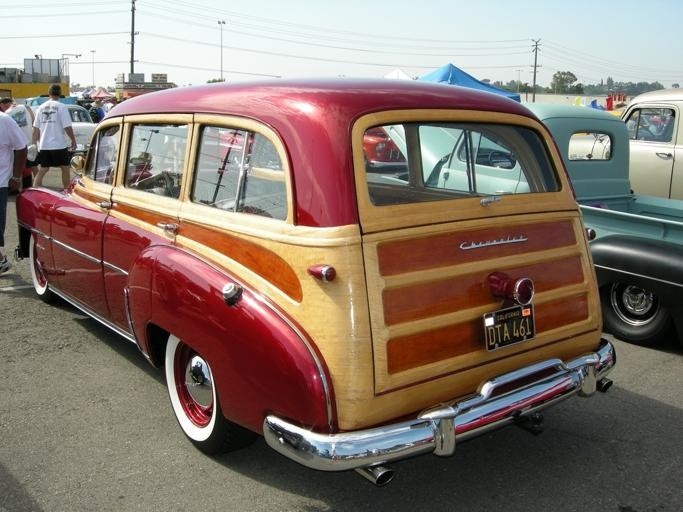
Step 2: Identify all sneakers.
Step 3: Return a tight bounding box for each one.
[0,256,14,274]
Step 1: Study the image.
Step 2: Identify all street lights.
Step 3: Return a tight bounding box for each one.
[90,50,97,85]
[217,21,224,82]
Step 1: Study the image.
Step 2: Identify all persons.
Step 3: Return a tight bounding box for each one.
[625,107,676,142]
[0,93,30,277]
[73,94,135,123]
[4,98,41,192]
[31,82,78,192]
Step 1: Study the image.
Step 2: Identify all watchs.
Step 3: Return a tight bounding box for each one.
[12,176,23,183]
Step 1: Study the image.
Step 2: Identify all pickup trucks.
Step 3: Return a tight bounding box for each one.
[366,98,683,341]
[568,90,683,203]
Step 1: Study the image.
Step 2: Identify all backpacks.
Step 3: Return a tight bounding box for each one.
[90,107,100,124]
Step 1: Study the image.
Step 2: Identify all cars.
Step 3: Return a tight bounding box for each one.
[19,84,619,485]
